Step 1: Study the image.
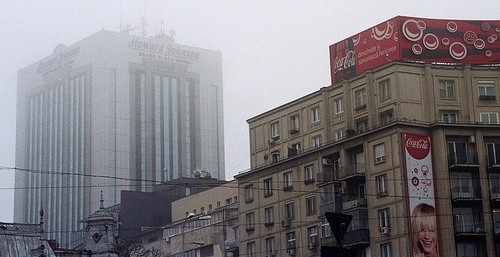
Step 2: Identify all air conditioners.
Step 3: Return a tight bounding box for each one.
[453,214,459,222]
[307,242,312,249]
[381,226,387,234]
[165,236,170,242]
[323,159,332,165]
[288,250,293,255]
[280,219,289,227]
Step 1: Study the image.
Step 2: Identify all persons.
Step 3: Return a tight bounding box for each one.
[411,203,440,257]
